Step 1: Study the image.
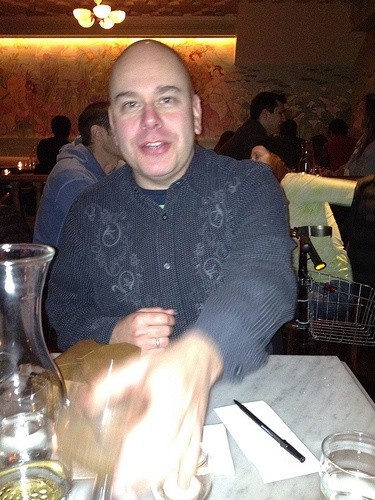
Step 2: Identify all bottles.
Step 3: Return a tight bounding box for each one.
[0,243,69,467]
[30,145,38,169]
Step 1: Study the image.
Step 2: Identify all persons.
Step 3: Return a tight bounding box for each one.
[44,39,297,492]
[35,90,375,355]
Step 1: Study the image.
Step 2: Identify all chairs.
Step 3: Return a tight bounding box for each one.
[0,173,50,230]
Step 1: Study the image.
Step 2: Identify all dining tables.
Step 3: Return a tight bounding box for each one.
[50,353,375,500]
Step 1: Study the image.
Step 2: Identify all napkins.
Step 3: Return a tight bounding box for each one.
[71,424,235,482]
[213,401,322,484]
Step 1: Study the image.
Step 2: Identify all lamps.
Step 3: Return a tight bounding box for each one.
[73,0,126,30]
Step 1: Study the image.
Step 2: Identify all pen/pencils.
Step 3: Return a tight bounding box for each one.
[233,399,305,463]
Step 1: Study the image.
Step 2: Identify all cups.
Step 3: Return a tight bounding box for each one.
[321,167,333,176]
[301,143,312,171]
[0,350,71,500]
[14,160,32,172]
[318,430,375,500]
[310,166,321,176]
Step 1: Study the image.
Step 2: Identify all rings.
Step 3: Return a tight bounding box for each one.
[154,337,160,350]
